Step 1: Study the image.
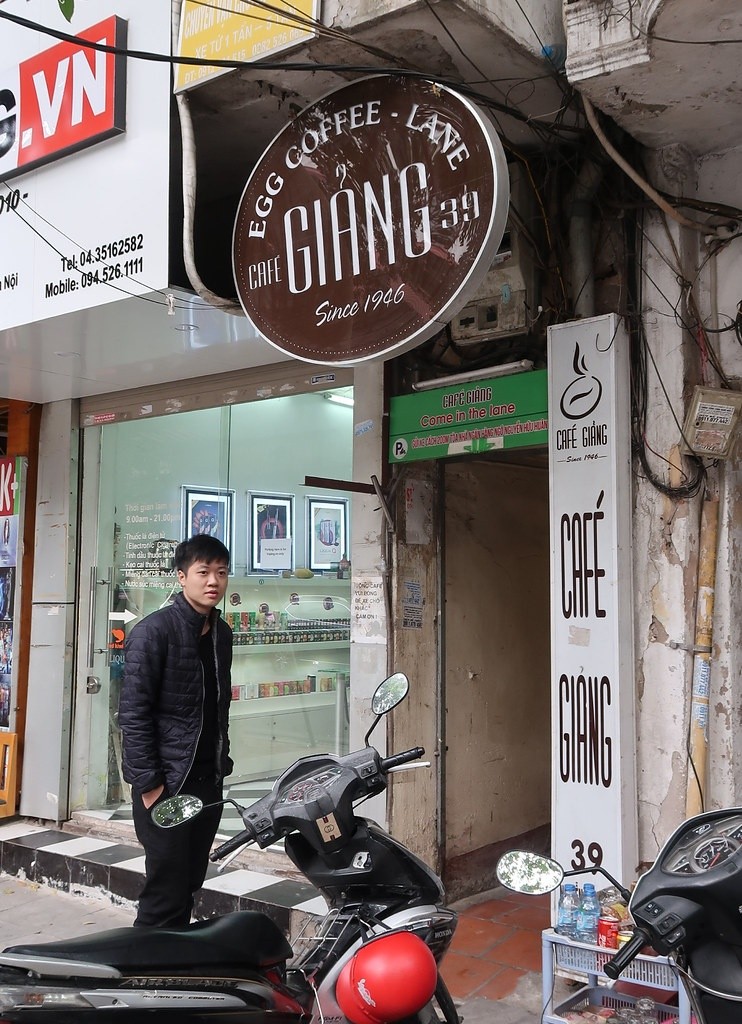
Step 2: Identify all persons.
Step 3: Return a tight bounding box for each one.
[117,533,234,931]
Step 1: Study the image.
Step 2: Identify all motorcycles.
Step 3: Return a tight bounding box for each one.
[0,669,476,1024]
[493,784,742,1024]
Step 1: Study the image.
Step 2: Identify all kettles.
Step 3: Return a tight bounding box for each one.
[148,537,177,575]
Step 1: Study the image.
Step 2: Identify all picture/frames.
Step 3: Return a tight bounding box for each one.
[307,498,350,572]
[250,493,296,574]
[184,487,234,578]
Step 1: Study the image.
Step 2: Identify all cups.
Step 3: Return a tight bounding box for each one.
[615,996,659,1024]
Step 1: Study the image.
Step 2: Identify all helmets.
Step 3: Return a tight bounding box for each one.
[336,903,438,1024]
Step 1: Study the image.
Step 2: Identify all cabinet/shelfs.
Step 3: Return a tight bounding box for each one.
[539,929,693,1024]
[129,572,353,786]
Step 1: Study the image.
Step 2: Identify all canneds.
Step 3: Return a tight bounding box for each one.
[596,916,618,948]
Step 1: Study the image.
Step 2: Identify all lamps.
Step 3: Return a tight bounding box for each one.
[57,351,82,362]
[174,322,201,334]
[323,393,355,409]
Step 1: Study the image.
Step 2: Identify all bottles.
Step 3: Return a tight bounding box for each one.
[230,618,350,645]
[116,582,126,613]
[599,887,618,916]
[337,553,350,578]
[583,883,594,890]
[577,889,597,946]
[316,668,350,692]
[557,884,578,939]
